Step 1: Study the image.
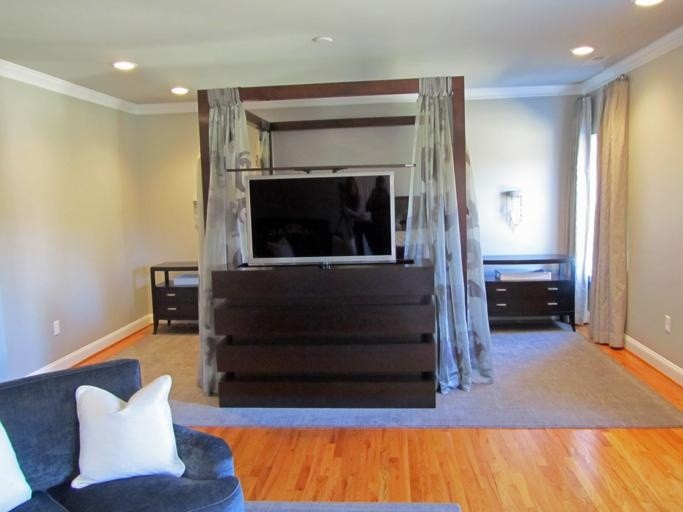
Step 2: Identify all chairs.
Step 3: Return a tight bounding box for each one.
[2,359,244,511]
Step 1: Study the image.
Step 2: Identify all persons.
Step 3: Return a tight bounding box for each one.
[343,176,390,254]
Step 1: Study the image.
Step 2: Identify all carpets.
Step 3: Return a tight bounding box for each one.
[105,320,682,429]
[244,501,461,512]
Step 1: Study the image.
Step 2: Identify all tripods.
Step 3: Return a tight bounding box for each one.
[332,205,355,239]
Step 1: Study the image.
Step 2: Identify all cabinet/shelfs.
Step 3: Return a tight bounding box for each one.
[149,261,201,333]
[483,254,576,332]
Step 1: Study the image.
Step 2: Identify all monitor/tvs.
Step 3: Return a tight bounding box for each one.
[245,170,397,268]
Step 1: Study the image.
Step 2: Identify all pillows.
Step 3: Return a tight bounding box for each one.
[70,376,188,490]
